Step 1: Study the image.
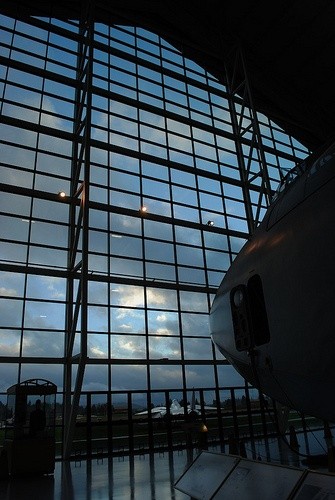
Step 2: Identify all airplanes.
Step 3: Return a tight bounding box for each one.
[139,397,217,423]
[210,147,335,421]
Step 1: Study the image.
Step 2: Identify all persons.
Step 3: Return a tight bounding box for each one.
[26,399,48,468]
